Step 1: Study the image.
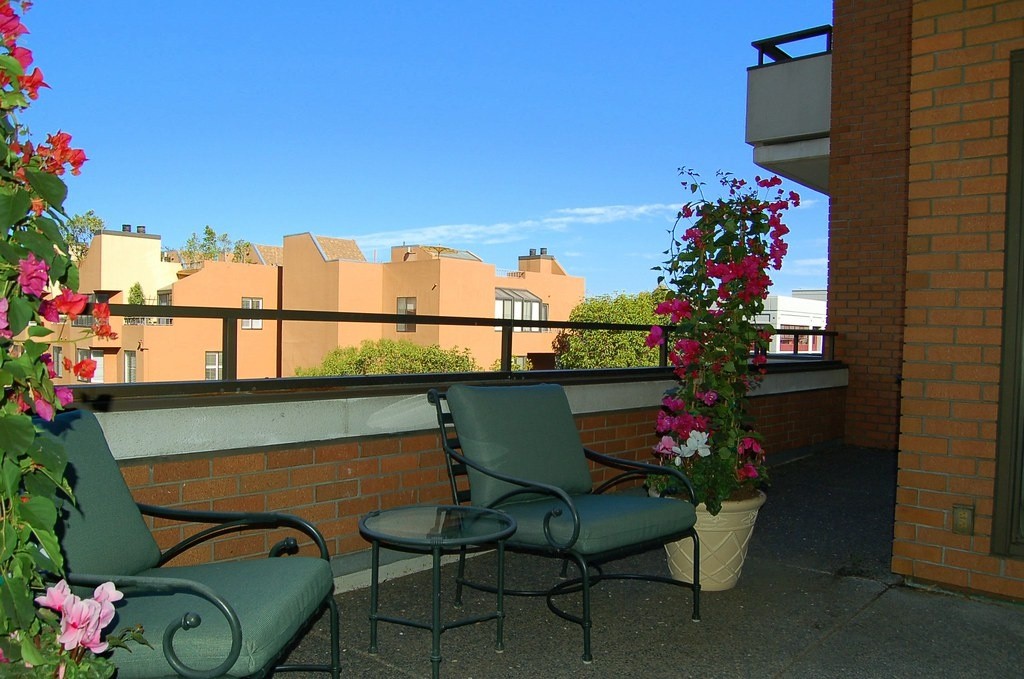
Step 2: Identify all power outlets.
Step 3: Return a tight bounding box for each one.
[952,504,975,536]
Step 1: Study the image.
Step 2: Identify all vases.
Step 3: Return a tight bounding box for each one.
[648,489,766,594]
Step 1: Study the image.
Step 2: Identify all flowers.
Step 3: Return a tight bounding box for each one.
[0,0,155,679]
[646,166,800,517]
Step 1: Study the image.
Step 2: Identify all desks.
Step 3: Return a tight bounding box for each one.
[358,503,517,679]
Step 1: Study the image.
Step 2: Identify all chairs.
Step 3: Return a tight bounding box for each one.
[16,408,339,679]
[428,384,701,664]
